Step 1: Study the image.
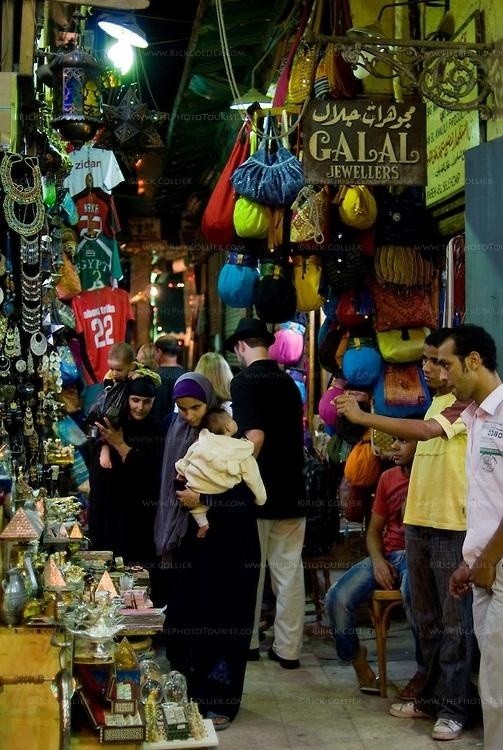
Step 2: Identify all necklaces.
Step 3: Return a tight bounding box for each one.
[0,151,42,206]
[22,302,41,334]
[20,267,42,302]
[19,235,39,266]
[2,183,45,237]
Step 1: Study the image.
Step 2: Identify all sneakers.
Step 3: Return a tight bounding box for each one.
[246,648,260,660]
[389,703,433,718]
[432,718,465,740]
[268,647,300,669]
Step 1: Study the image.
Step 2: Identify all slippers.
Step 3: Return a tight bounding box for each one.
[400,672,428,700]
[206,711,230,730]
[360,676,381,693]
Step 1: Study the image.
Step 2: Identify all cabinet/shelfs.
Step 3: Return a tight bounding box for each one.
[0,626,75,750]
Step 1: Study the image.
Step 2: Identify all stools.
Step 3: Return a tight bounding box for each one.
[366,589,402,698]
[302,554,357,623]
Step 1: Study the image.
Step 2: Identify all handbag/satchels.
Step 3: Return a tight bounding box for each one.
[371,281,436,332]
[318,287,371,326]
[292,255,325,312]
[373,364,432,417]
[217,247,259,308]
[272,1,314,107]
[371,430,396,460]
[324,231,378,294]
[315,1,363,101]
[254,258,297,324]
[233,111,272,239]
[335,329,351,369]
[288,1,328,105]
[332,185,377,229]
[344,427,381,488]
[376,327,433,363]
[51,306,79,387]
[319,321,342,375]
[267,313,306,366]
[268,185,329,251]
[337,390,371,446]
[55,252,82,301]
[56,382,83,413]
[374,185,441,246]
[319,377,348,428]
[374,247,434,295]
[342,325,381,386]
[52,408,88,452]
[201,119,254,246]
[229,110,309,208]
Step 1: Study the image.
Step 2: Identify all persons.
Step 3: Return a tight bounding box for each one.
[223,317,307,669]
[438,322,503,750]
[325,433,426,701]
[154,372,262,732]
[333,327,478,741]
[175,407,267,537]
[80,375,172,608]
[150,334,194,419]
[134,342,160,371]
[171,352,233,419]
[87,342,161,469]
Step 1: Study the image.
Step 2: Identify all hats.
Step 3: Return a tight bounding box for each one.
[156,336,182,351]
[225,318,275,353]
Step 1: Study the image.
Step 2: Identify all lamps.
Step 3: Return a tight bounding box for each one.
[33,49,104,146]
[345,0,450,79]
[97,9,148,49]
[229,9,302,110]
